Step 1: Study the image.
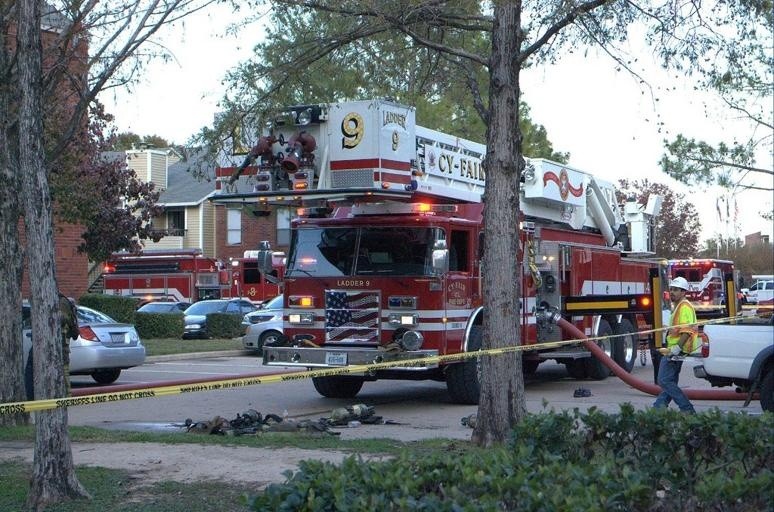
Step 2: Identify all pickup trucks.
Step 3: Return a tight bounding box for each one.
[692,314,774,415]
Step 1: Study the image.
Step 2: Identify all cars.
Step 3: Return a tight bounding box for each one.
[135,292,284,356]
[18,297,148,388]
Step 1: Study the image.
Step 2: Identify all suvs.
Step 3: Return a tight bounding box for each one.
[741,279,774,305]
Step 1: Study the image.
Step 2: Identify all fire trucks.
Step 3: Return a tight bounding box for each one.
[209,94,666,407]
[666,257,745,320]
[100,240,320,306]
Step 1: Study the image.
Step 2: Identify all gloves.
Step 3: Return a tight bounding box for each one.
[670,344,681,356]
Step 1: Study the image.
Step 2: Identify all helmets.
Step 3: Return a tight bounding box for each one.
[668,276,689,291]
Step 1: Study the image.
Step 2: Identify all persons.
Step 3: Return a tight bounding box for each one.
[58,291,77,398]
[651,275,703,415]
